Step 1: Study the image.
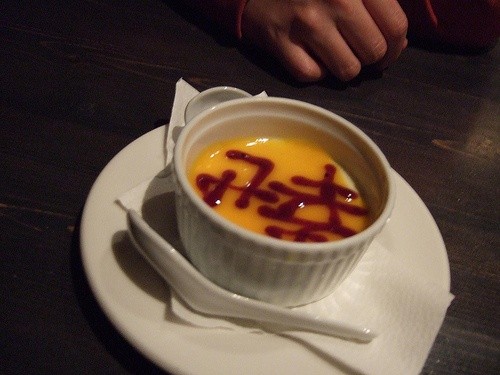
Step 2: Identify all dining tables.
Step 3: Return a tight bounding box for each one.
[0,0,500,375]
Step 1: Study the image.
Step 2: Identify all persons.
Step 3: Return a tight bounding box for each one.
[176,1,500,86]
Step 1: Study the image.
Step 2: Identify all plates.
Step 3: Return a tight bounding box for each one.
[80,110,450,375]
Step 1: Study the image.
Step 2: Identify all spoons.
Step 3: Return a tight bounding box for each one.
[184,84,256,126]
[125,210,376,344]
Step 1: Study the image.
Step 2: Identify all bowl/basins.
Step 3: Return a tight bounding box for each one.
[174,96,395,311]
